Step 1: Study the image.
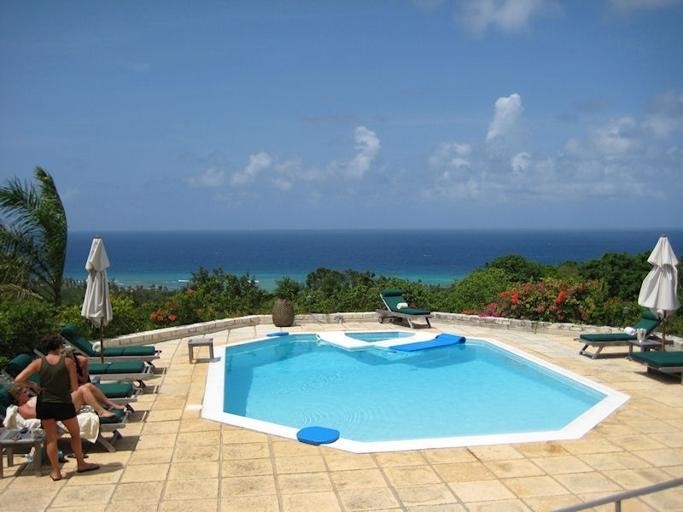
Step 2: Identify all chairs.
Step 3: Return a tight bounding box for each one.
[0,324,162,476]
[375,289,431,330]
[573,309,683,384]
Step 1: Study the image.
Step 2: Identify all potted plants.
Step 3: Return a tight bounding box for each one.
[272,273,301,327]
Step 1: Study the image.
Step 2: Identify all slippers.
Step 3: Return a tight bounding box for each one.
[50,472,61,481]
[78,464,99,472]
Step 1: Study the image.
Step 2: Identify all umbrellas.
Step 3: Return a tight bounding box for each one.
[637,234,682,350]
[80,233,112,364]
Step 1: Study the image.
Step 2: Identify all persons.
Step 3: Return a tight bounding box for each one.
[14,333,98,479]
[8,378,126,419]
[60,345,91,386]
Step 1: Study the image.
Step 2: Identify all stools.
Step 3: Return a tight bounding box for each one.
[188,338,213,364]
